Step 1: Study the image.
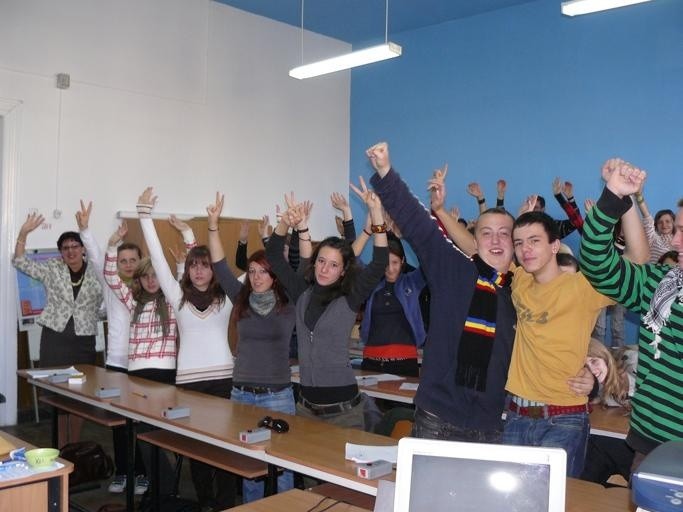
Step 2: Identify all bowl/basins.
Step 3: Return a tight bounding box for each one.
[23,446,60,468]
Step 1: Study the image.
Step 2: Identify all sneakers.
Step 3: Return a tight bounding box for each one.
[108,474,127,494]
[134,474,149,496]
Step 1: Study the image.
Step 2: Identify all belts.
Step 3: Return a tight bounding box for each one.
[233,381,293,395]
[298,393,361,415]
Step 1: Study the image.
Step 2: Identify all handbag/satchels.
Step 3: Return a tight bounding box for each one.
[58,439,115,487]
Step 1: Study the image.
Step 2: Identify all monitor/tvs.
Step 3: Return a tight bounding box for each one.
[393,436,569,512]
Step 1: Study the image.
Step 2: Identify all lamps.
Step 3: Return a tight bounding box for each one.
[558,0,657,22]
[287,1,404,82]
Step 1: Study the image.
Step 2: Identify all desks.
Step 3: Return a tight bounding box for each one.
[109,389,302,512]
[1,430,74,512]
[19,363,175,509]
[291,359,631,440]
[218,426,636,512]
